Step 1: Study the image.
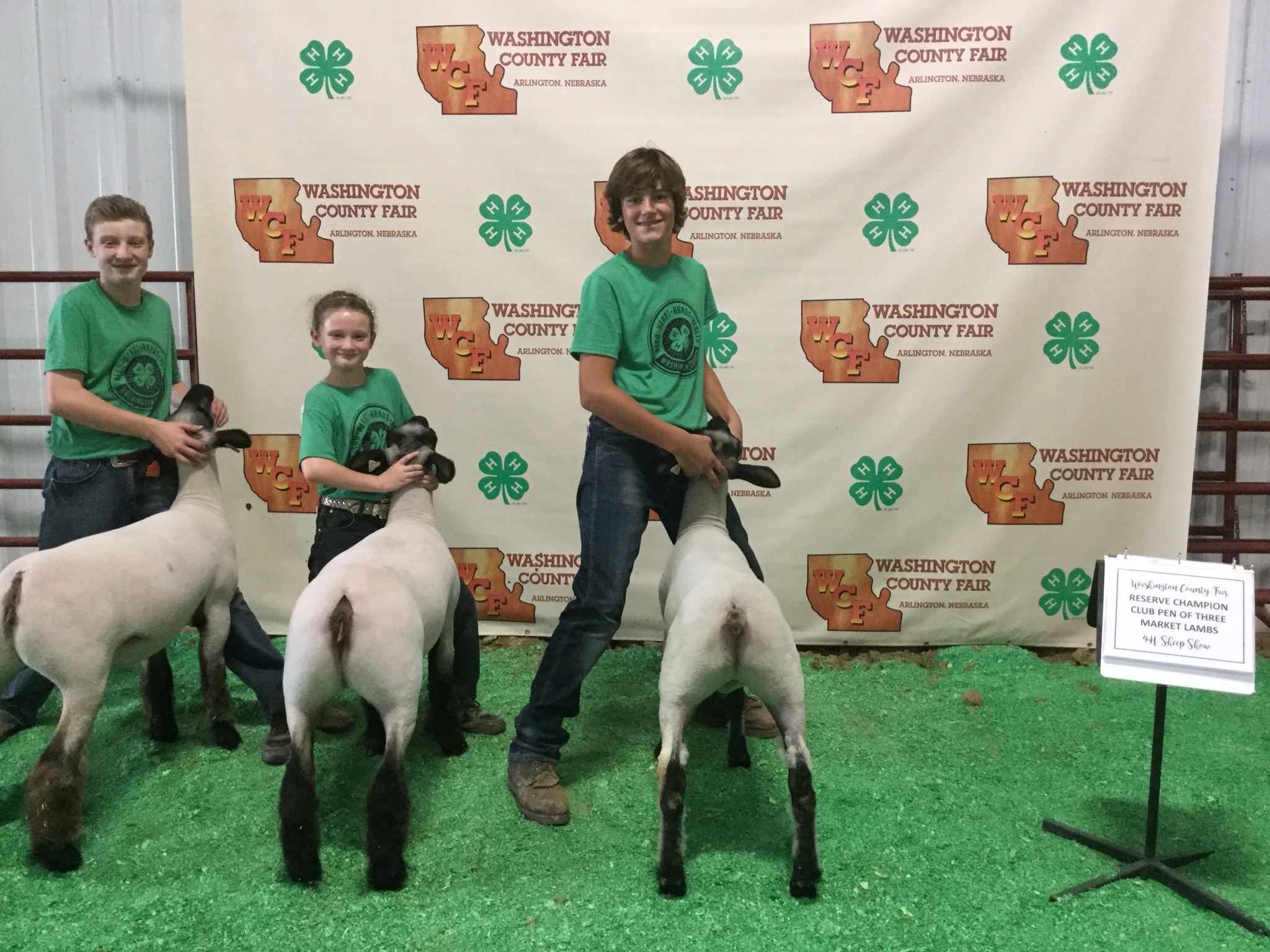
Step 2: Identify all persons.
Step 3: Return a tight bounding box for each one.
[294,291,508,739]
[503,147,783,828]
[0,192,299,766]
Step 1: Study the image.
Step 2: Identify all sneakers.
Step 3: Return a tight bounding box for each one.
[315,697,354,729]
[261,716,292,765]
[425,700,507,734]
[507,761,570,826]
[701,689,779,739]
[0,706,35,744]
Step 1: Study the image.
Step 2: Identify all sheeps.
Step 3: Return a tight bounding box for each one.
[648,410,827,909]
[0,380,256,878]
[271,413,471,896]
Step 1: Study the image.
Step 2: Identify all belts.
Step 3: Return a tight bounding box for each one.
[111,448,153,468]
[322,495,389,519]
